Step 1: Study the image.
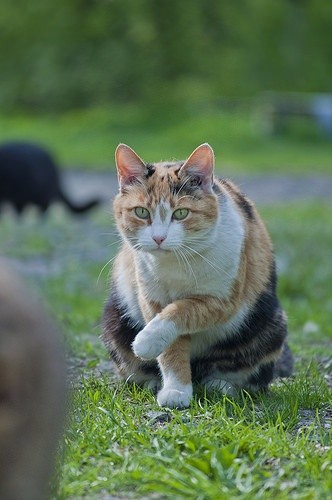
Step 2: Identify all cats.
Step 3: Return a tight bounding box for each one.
[0,143,101,225]
[97,142,294,409]
